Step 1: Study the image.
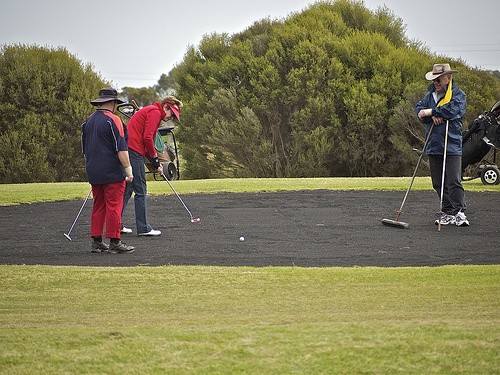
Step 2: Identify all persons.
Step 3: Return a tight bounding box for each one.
[120,96,183,236]
[81,89,135,254]
[415,64,470,227]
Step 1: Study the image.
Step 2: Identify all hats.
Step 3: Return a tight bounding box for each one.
[91,90,125,107]
[165,103,180,124]
[425,63,458,81]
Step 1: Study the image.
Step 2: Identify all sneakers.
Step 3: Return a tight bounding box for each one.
[120,227,133,233]
[435,215,456,225]
[109,240,135,254]
[454,211,470,226]
[91,241,109,252]
[137,229,162,236]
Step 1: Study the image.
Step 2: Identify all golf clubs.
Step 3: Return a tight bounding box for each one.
[64,190,92,241]
[163,174,200,223]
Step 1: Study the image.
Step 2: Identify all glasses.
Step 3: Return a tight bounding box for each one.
[433,76,447,82]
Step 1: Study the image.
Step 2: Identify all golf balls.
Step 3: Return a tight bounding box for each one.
[126,176,129,181]
[239,237,244,242]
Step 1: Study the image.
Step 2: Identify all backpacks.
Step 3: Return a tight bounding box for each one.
[461,111,500,170]
